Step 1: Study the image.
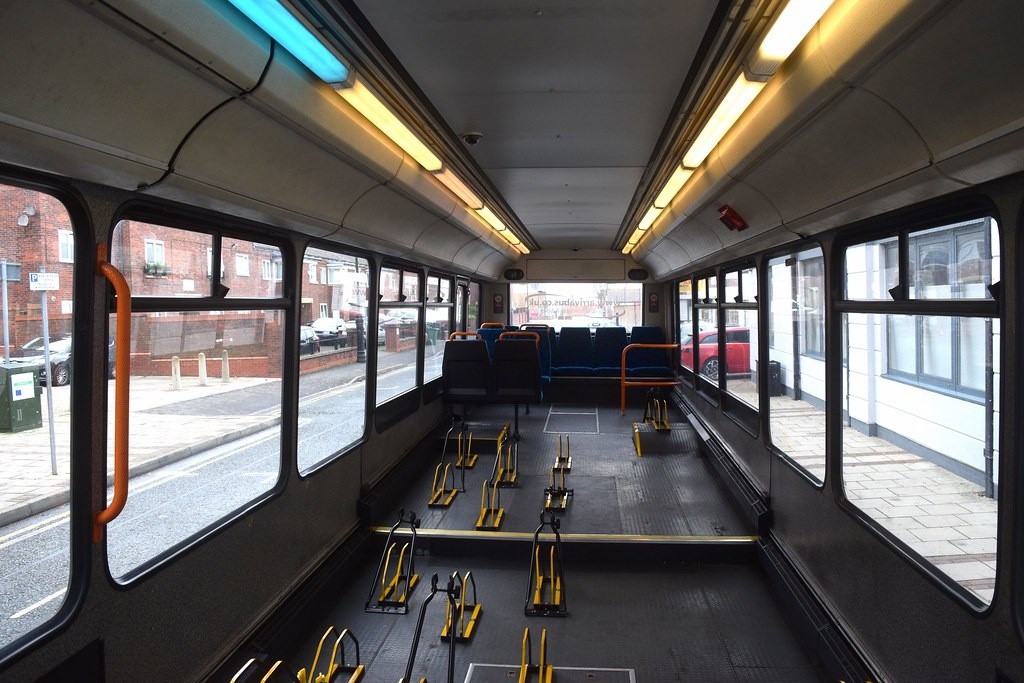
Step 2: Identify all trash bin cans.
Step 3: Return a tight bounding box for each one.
[755,360,782,397]
[426,328,439,345]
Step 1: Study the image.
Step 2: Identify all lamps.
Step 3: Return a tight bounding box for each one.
[622,0,835,257]
[225,0,532,255]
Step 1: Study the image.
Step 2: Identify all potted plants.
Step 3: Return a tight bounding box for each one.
[145,262,171,275]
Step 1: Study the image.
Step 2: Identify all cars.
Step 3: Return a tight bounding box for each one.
[0,332,117,387]
[345,308,419,346]
[307,317,347,350]
[681,320,718,345]
[681,328,751,381]
[299,325,320,355]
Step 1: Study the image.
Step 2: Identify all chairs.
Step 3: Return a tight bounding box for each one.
[439,322,676,441]
